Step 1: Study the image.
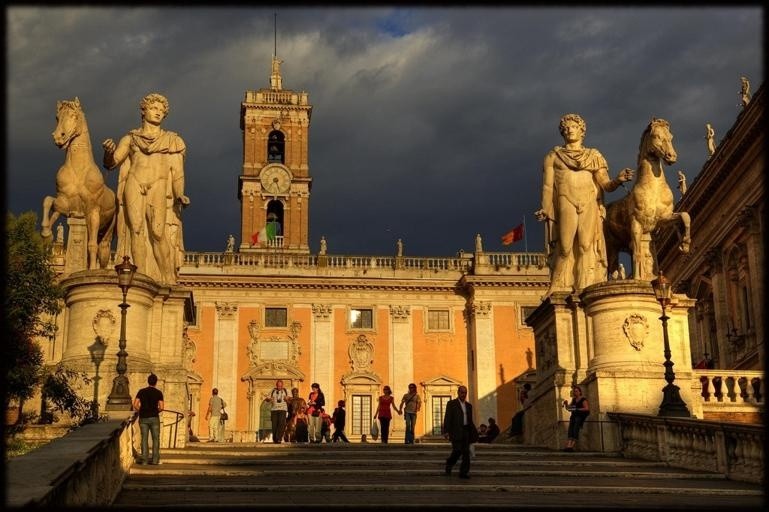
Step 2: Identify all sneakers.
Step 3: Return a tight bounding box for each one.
[559,446,574,453]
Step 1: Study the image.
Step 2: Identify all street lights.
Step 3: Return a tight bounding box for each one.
[104,255,138,411]
[650,270,688,416]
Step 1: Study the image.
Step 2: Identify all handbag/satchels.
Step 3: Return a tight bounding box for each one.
[221,399,228,420]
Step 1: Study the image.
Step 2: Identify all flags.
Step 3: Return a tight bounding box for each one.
[253,218,280,246]
[500,222,527,247]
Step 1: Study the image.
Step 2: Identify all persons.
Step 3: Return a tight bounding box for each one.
[320,234,328,253]
[737,75,753,106]
[443,385,476,480]
[562,387,589,448]
[477,416,501,443]
[704,122,718,157]
[676,170,687,197]
[396,238,404,257]
[374,383,422,443]
[270,49,284,73]
[102,90,192,285]
[205,388,226,442]
[224,233,235,251]
[534,113,636,296]
[264,379,351,444]
[474,232,484,251]
[134,374,164,466]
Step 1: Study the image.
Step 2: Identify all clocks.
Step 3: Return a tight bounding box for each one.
[258,163,293,195]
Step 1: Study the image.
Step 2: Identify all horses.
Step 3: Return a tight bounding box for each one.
[41,96,116,270]
[603,116,691,279]
